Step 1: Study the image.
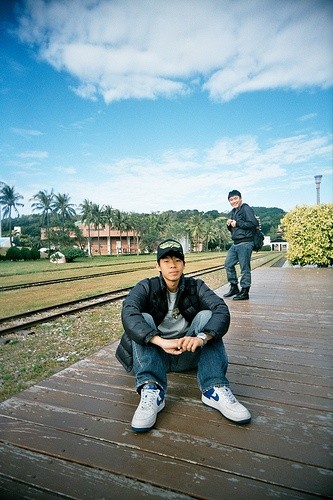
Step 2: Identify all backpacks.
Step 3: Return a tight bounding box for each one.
[241,204,264,250]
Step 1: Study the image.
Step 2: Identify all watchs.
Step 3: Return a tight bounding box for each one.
[196,333,208,344]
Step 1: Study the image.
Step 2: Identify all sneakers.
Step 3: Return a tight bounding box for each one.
[201,385,252,423]
[131,384,165,431]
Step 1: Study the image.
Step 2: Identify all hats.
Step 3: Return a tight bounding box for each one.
[157,239,184,261]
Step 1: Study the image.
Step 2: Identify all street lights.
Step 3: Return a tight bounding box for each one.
[314,175,323,205]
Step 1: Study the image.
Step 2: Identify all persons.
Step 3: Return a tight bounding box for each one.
[223,190,260,301]
[117,239,253,431]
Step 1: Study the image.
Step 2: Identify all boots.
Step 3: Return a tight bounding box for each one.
[233,287,249,300]
[224,284,239,297]
